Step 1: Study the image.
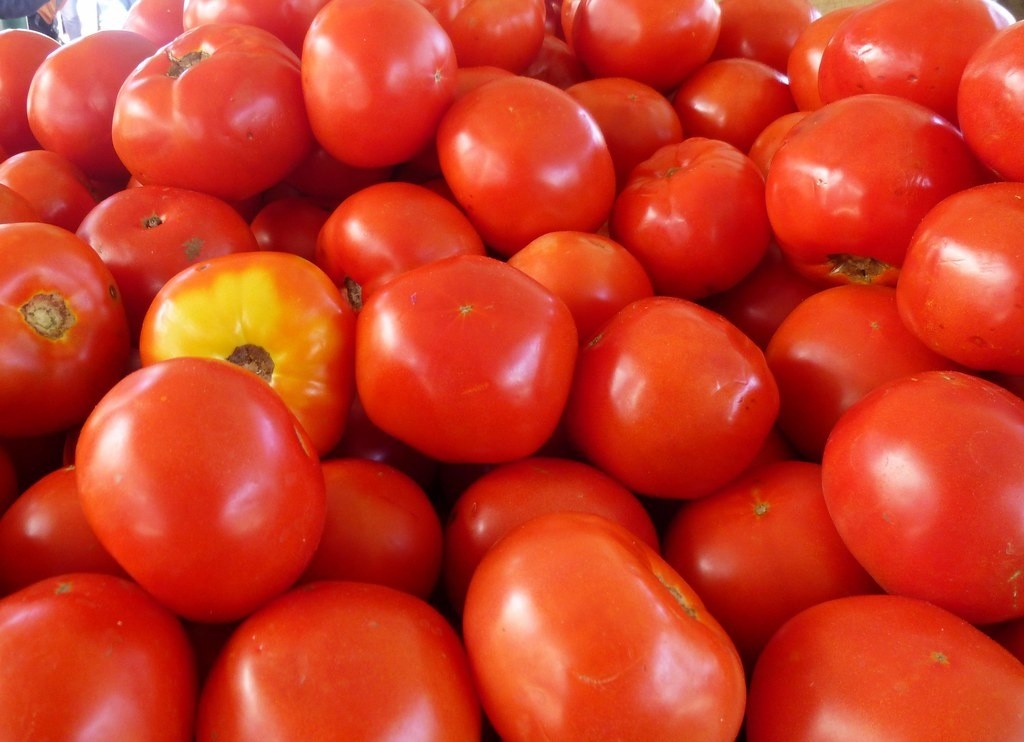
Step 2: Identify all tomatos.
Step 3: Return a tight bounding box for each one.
[1,0,1023,742]
